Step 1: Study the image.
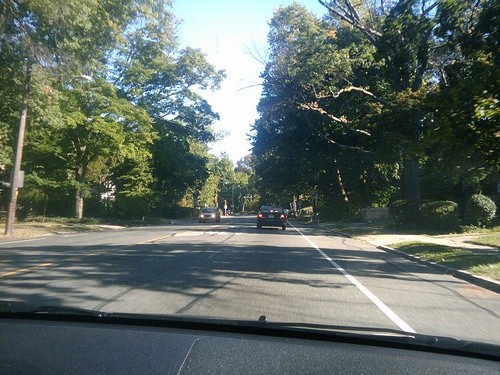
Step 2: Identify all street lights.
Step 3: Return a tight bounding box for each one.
[4,49,93,233]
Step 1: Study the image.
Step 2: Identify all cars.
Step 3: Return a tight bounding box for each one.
[256,205,287,231]
[1,304,500,375]
[198,207,220,223]
